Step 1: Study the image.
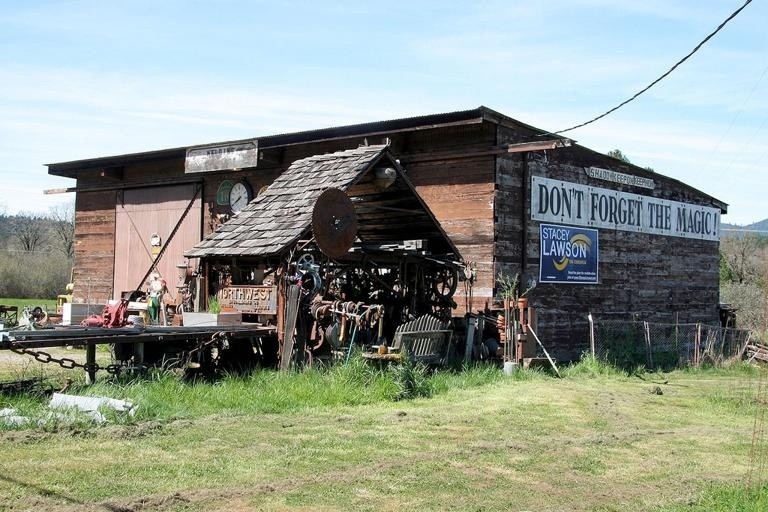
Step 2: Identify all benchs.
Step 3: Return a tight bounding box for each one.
[360,315,452,367]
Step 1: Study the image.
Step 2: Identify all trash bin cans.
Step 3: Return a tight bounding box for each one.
[56,295,72,315]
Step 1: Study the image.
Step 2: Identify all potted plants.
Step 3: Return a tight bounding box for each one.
[496,271,522,379]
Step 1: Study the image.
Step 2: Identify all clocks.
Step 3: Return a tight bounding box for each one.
[229,183,248,216]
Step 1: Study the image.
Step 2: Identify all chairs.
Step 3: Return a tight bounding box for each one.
[57,283,74,308]
